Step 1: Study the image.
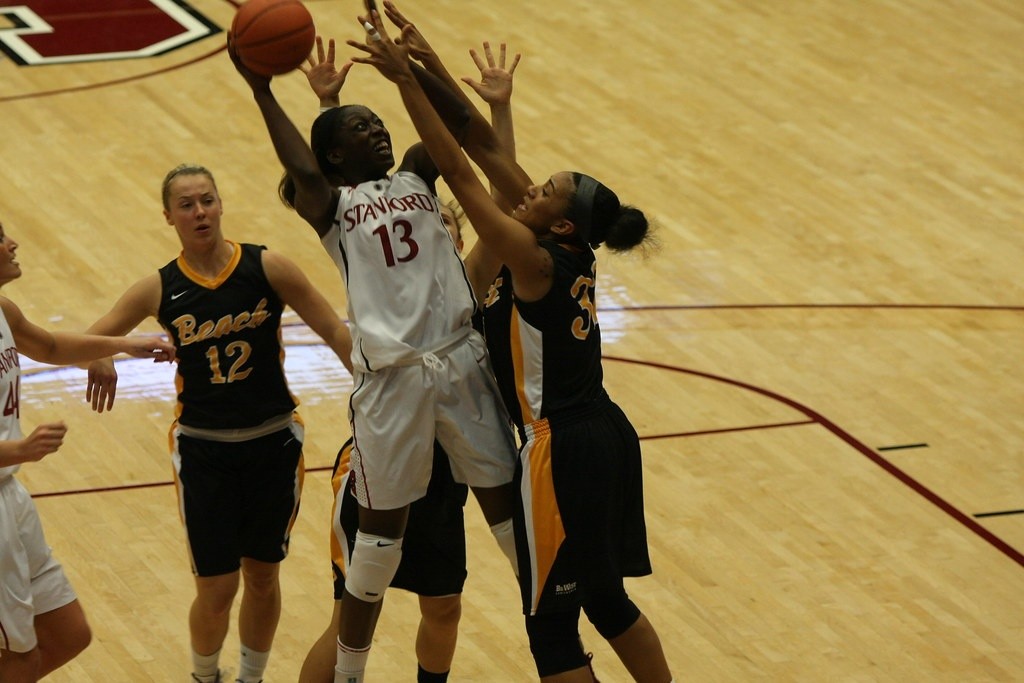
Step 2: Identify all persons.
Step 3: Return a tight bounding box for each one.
[73,165,354,681]
[290,28,516,678]
[347,0,676,681]
[1,223,179,681]
[223,0,599,683]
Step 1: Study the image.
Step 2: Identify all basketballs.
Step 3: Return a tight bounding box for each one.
[231,0,317,77]
